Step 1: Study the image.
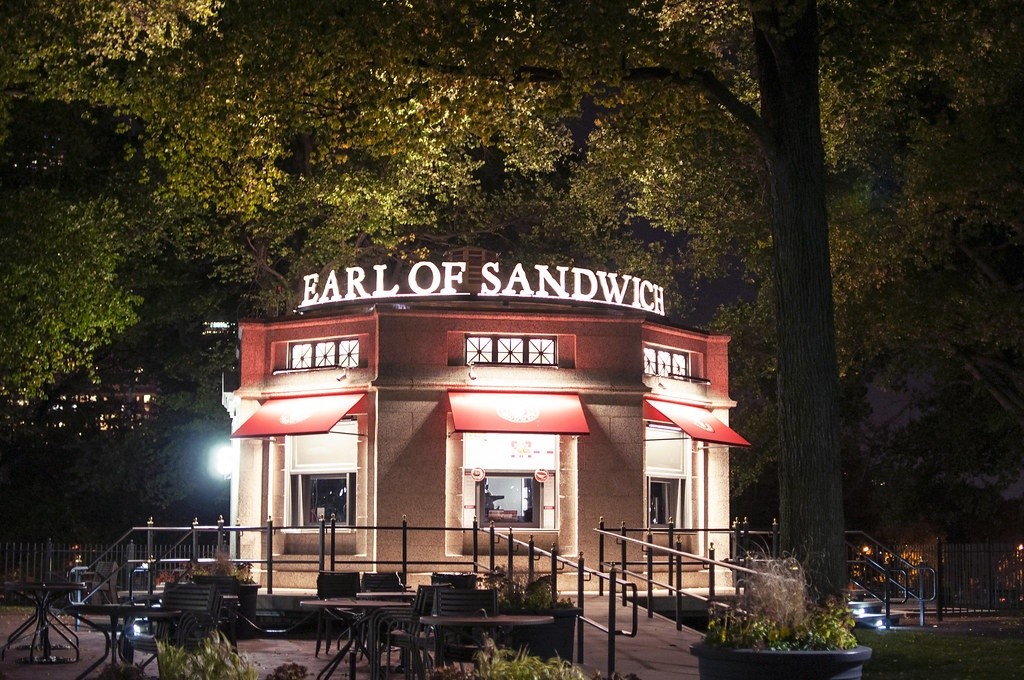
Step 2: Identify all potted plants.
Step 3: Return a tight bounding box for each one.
[192,562,262,638]
[690,536,872,680]
[474,560,584,663]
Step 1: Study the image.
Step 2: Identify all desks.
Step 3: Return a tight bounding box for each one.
[63,604,183,680]
[420,615,554,649]
[3,584,87,664]
[300,600,411,680]
[357,592,417,602]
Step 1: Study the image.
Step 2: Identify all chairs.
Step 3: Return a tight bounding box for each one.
[82,561,119,604]
[315,570,498,680]
[121,575,242,674]
